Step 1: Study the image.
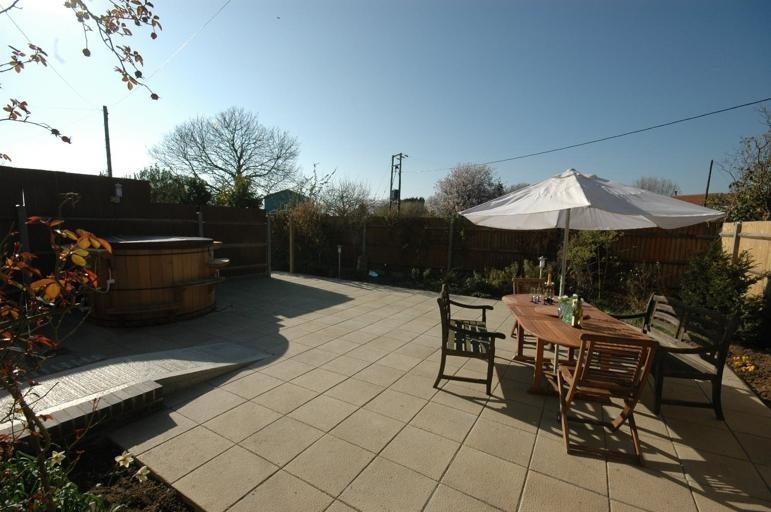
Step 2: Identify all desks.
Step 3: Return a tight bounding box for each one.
[499,292,658,392]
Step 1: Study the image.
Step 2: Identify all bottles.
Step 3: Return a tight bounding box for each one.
[572,294,584,329]
[545,272,553,304]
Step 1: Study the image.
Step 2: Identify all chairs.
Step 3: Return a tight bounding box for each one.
[554,331,656,463]
[511,276,557,349]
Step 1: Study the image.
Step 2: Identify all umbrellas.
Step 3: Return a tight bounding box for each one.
[456,167,728,301]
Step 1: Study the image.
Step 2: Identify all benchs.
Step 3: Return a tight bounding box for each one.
[610,291,737,420]
[434,284,507,394]
